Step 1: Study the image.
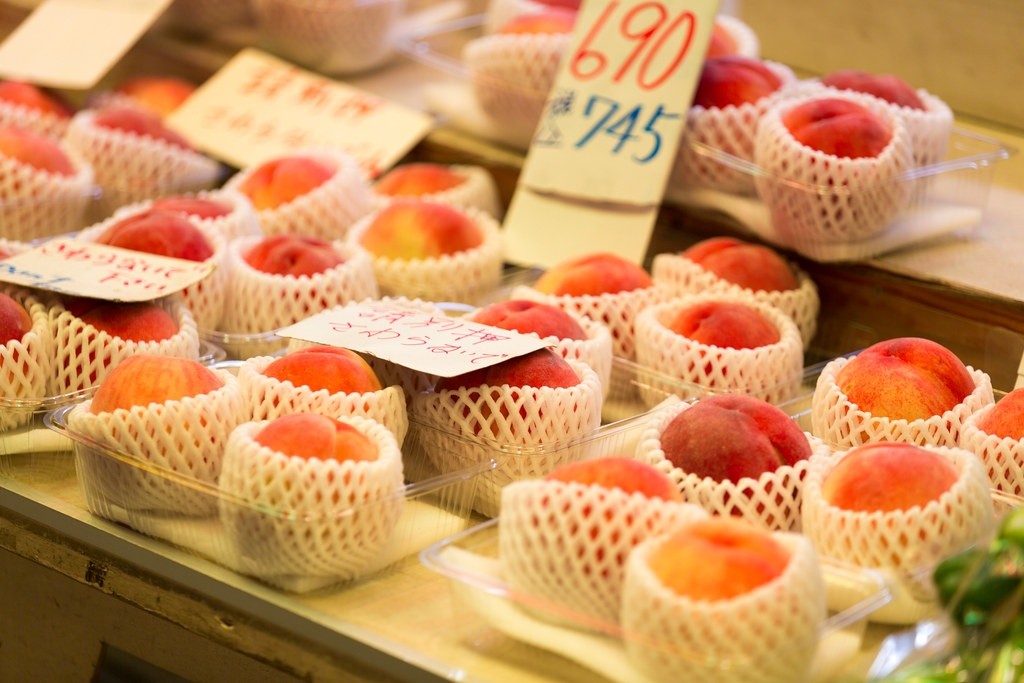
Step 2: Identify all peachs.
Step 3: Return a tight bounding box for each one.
[0,0,1024,683]
